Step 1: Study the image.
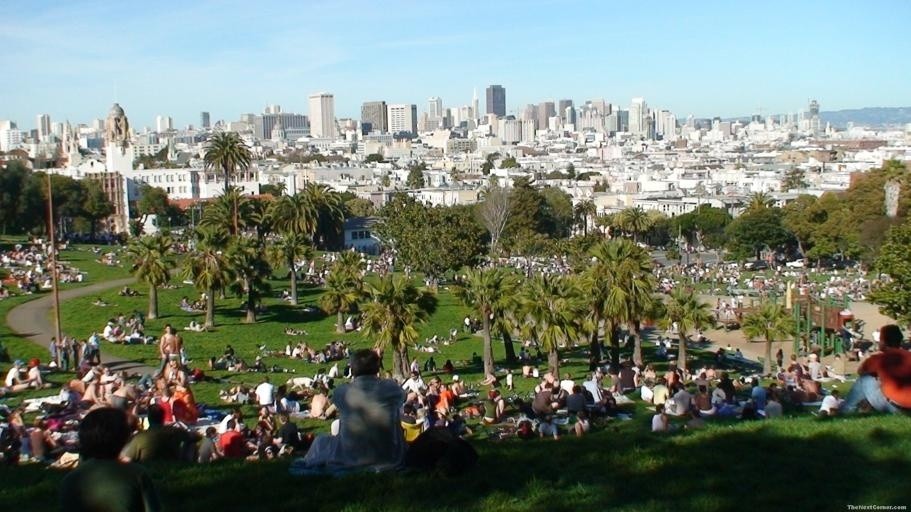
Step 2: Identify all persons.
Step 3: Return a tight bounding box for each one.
[0,227,911,511]
